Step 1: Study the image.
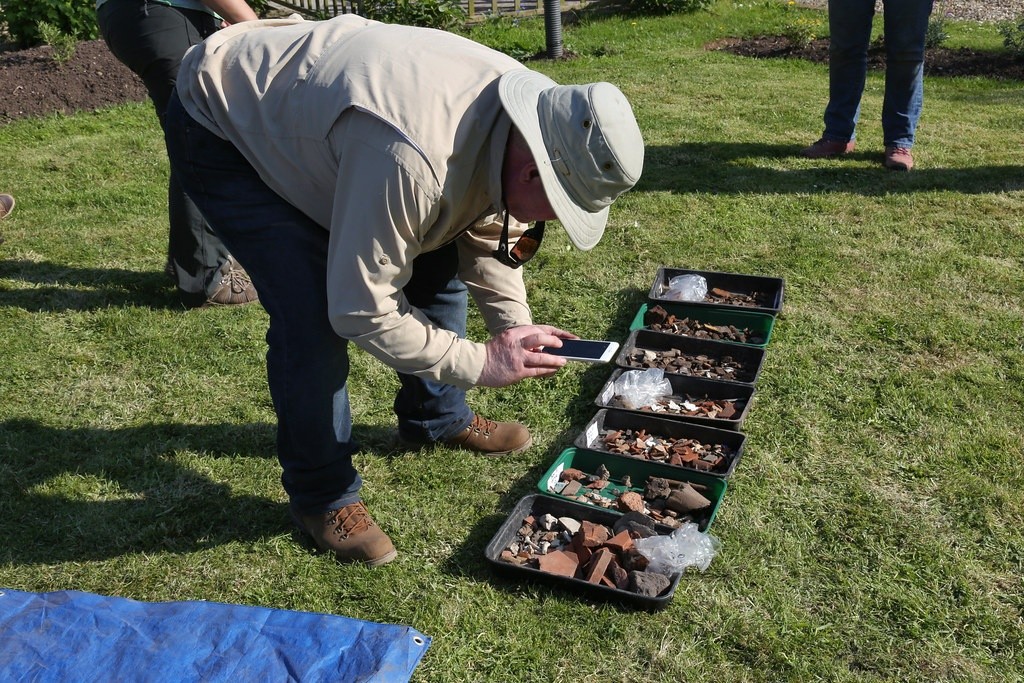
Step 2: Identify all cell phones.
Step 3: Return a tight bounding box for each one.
[538,338,620,363]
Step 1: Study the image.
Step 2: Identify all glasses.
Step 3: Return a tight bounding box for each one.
[496,197,548,271]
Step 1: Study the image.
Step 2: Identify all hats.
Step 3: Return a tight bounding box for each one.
[496,66,646,252]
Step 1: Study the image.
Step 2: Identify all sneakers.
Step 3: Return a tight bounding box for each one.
[291,491,397,571]
[209,268,259,305]
[399,414,533,457]
[884,147,913,171]
[800,133,855,158]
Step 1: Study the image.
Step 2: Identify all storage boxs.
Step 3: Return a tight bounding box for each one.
[574,407,748,478]
[614,329,767,386]
[595,368,757,432]
[629,301,774,349]
[537,446,727,534]
[648,267,786,316]
[484,493,684,610]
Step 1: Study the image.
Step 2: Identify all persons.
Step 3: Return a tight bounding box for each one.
[93,0,257,304]
[161,12,644,566]
[798,0,935,170]
